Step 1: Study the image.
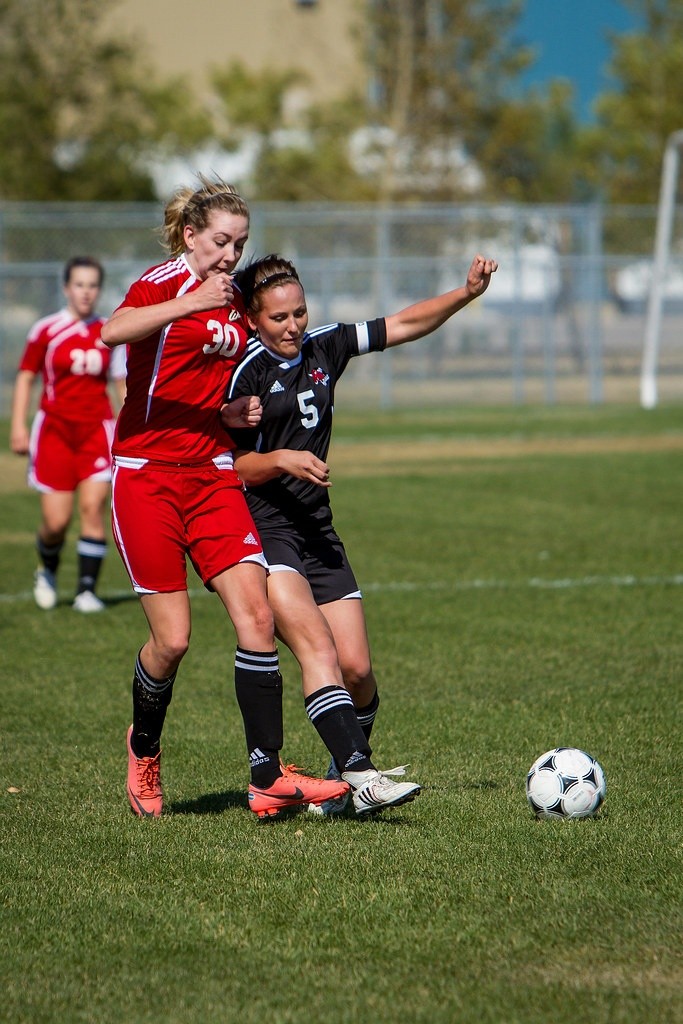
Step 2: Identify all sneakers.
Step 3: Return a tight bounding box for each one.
[248,758,348,817]
[309,751,349,815]
[73,589,105,614]
[352,763,422,818]
[126,723,163,820]
[32,565,58,609]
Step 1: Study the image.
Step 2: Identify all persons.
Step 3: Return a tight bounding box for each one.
[225,255,499,816]
[9,256,127,612]
[100,185,350,819]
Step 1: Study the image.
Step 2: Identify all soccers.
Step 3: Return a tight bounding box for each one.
[524,746,610,820]
